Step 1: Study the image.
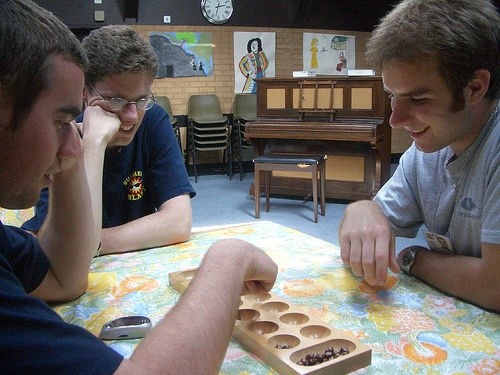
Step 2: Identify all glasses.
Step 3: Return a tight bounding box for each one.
[88,81,157,110]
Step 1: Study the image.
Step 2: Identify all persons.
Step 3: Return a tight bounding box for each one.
[338,0,500,315]
[0,0,278,375]
[20,24,196,258]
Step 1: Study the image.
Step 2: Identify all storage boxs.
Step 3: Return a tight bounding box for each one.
[348,69,376,76]
[292,71,316,77]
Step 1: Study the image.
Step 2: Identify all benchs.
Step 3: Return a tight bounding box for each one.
[253,154,328,223]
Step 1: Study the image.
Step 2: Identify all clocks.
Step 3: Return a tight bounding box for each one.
[201,0,234,24]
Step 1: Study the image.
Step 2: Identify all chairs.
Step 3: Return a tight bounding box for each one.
[151,96,183,156]
[187,94,233,183]
[232,94,258,181]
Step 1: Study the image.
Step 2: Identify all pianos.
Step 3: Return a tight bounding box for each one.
[244,74,393,202]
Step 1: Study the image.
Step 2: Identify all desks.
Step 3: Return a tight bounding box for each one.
[47,221,500,374]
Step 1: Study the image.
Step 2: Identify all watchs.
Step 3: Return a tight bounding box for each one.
[95,242,102,257]
[398,246,430,277]
